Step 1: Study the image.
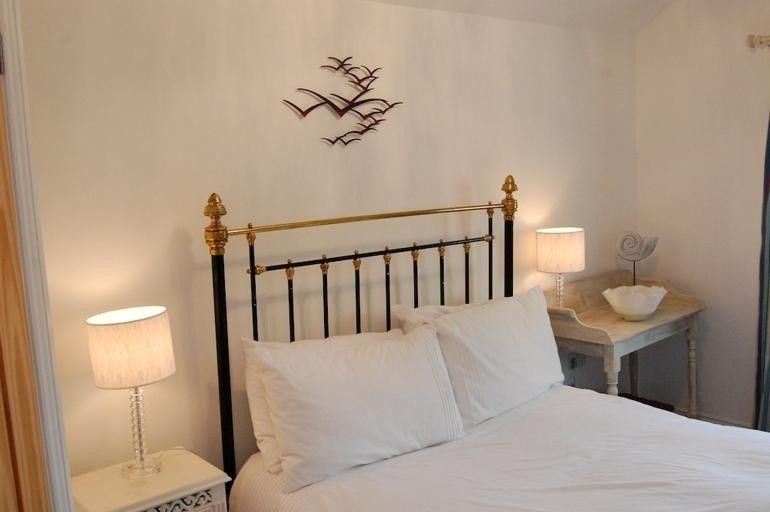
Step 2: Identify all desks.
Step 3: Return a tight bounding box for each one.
[543,268,706,420]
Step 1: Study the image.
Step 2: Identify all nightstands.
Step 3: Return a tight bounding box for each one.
[70,446,232,512]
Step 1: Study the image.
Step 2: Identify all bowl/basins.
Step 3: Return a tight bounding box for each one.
[601,284,668,323]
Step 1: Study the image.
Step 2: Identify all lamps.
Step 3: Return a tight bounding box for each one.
[85,305,177,479]
[535,227,587,308]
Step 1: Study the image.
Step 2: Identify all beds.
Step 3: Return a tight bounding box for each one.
[203,174,769,511]
